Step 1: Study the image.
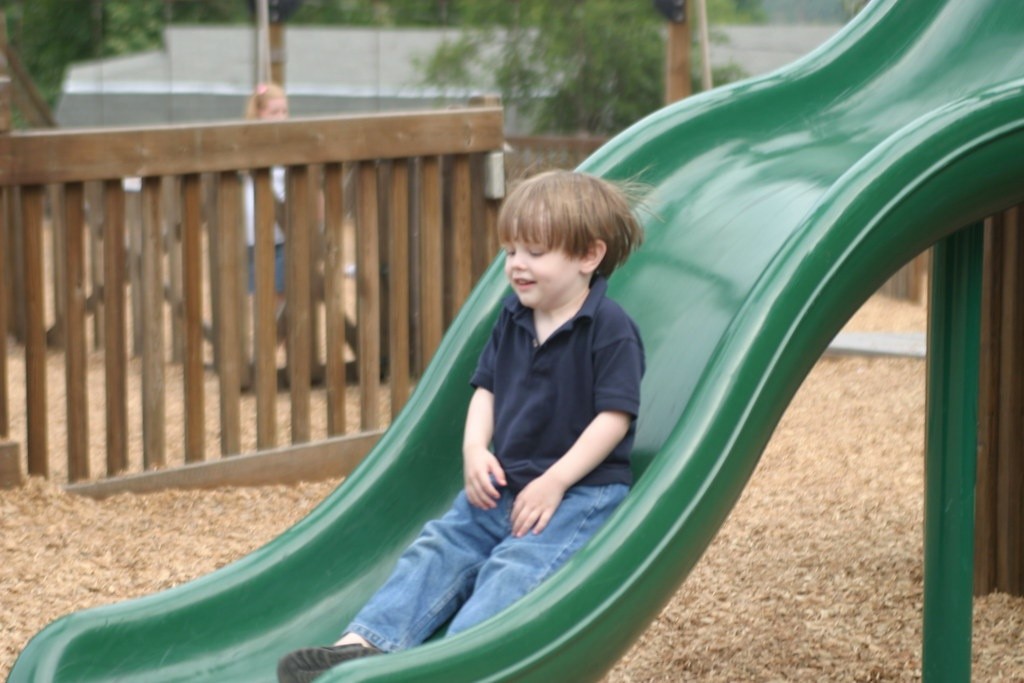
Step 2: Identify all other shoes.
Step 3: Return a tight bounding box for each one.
[277,644,383,683]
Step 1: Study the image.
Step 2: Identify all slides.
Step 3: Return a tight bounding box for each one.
[5,0,1024,683]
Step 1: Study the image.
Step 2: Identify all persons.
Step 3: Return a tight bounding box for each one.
[242,84,289,370]
[277,170,645,683]
